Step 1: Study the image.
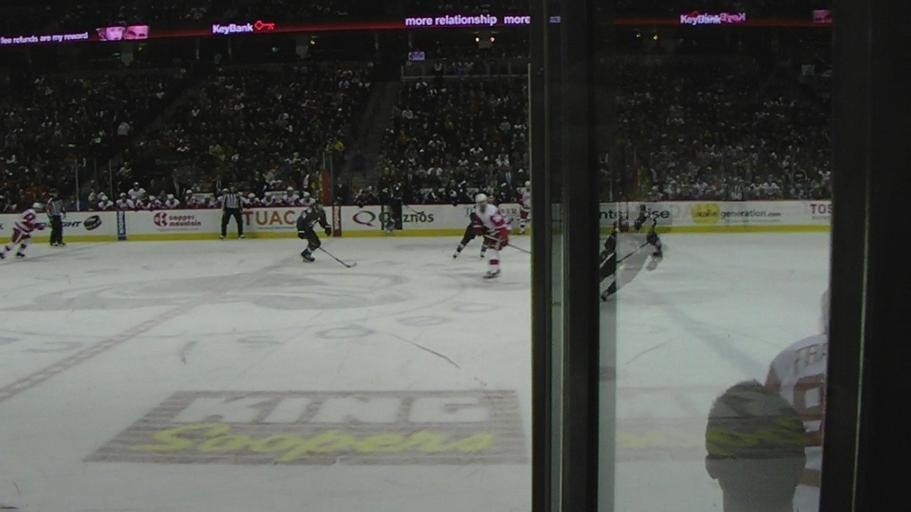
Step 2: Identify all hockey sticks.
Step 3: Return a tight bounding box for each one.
[318,246,360,268]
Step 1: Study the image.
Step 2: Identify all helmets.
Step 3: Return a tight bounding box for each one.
[33,202,43,209]
[310,198,322,207]
[525,181,531,187]
[475,193,487,205]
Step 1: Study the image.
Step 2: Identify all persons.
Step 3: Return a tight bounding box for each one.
[42,189,68,250]
[297,199,332,262]
[221,186,246,238]
[474,194,512,276]
[453,208,491,258]
[0,2,533,213]
[614,62,834,202]
[762,286,834,511]
[599,215,667,300]
[703,380,807,511]
[0,202,50,258]
[515,180,532,235]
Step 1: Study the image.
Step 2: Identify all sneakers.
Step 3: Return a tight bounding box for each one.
[516,230,526,235]
[480,247,487,257]
[301,249,315,261]
[50,241,66,247]
[601,287,614,302]
[0,253,5,259]
[482,268,501,278]
[219,234,247,239]
[16,252,25,257]
[452,247,462,258]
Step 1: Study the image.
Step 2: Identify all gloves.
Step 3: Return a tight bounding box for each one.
[647,260,658,270]
